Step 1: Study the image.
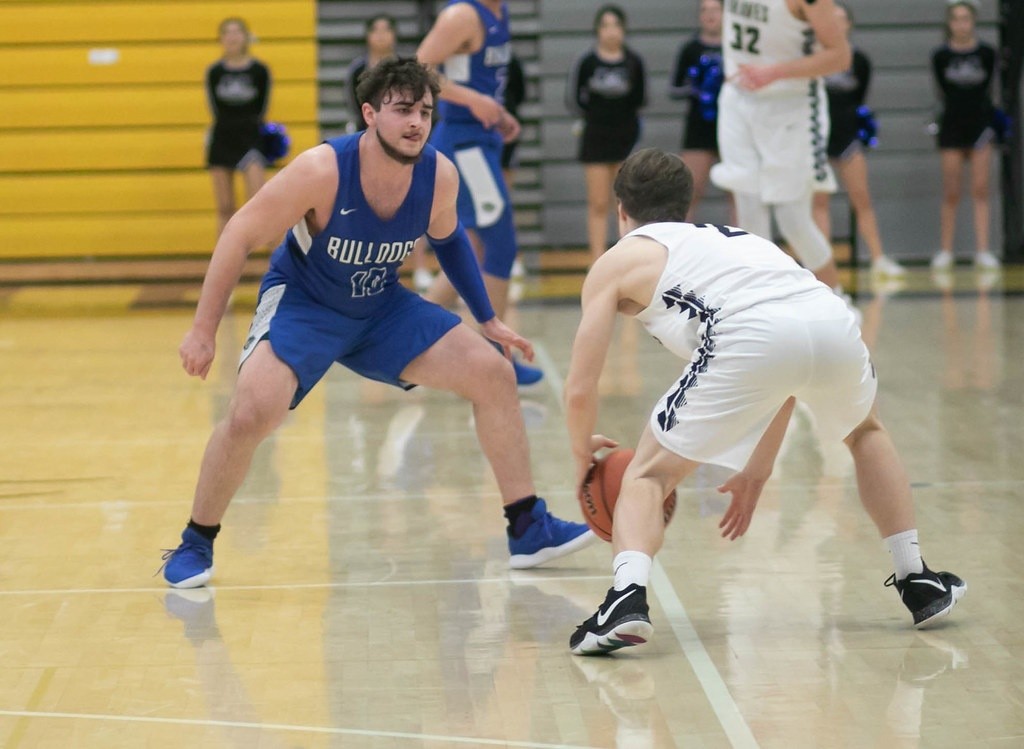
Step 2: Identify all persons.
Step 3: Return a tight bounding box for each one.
[159,57,598,590]
[929,1,1006,268]
[416,0,543,387]
[348,14,432,291]
[667,0,907,324]
[204,18,272,308]
[566,6,647,266]
[564,147,967,655]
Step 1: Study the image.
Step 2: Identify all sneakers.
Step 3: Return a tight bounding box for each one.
[973,247,1002,273]
[892,560,968,631]
[931,250,955,272]
[164,528,215,590]
[511,356,544,386]
[869,253,910,279]
[569,583,657,662]
[506,499,600,571]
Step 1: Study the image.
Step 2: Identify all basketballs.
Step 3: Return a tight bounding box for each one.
[579,443,681,545]
